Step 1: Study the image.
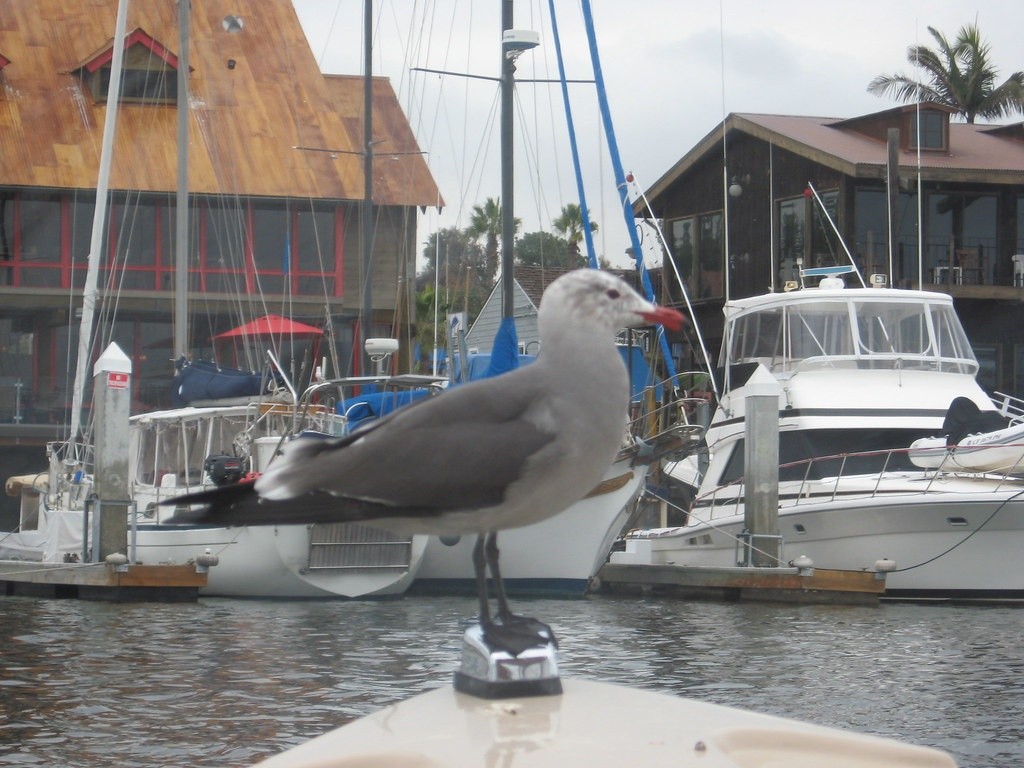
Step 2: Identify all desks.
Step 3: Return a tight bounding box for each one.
[933,267,962,285]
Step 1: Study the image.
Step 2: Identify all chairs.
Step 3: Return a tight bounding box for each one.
[1012,254,1024,287]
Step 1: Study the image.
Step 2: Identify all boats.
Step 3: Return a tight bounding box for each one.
[623,264,1024,605]
[0,1,426,598]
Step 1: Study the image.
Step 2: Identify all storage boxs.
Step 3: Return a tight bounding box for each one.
[254,435,289,473]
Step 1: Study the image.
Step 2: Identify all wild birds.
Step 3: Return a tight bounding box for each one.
[156,266,692,657]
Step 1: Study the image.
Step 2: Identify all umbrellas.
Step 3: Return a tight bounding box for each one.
[208,314,324,372]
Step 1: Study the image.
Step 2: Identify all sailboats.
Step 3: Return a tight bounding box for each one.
[173,0,710,602]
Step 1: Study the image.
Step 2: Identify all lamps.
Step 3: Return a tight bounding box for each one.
[729,173,742,196]
